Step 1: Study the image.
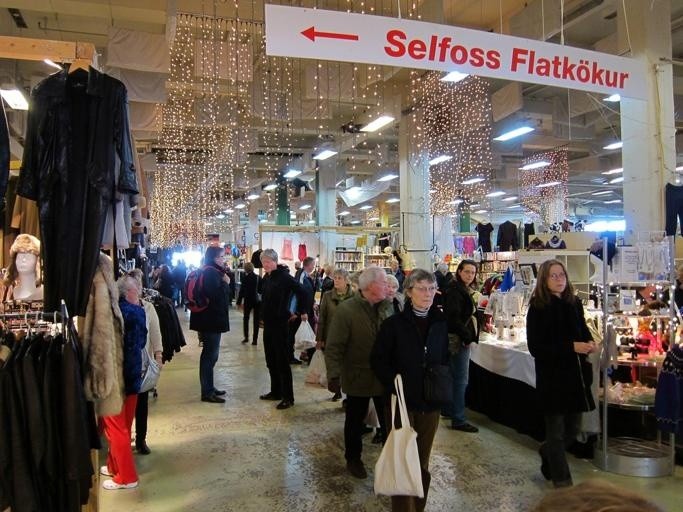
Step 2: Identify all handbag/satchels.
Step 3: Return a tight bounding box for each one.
[576,352,602,434]
[372,374,424,499]
[138,345,162,394]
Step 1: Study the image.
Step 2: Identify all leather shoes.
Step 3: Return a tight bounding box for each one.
[212,387,226,396]
[276,400,295,410]
[201,394,225,403]
[258,392,283,401]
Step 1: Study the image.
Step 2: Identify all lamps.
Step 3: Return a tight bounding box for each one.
[290,178,312,200]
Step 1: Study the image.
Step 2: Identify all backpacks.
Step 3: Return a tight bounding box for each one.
[185,269,211,312]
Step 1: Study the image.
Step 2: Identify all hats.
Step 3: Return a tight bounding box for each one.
[3,230,44,287]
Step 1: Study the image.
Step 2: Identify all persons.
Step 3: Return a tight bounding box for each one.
[131,269,165,454]
[371,271,449,512]
[388,262,405,285]
[154,264,174,296]
[445,261,479,432]
[260,249,309,409]
[526,259,598,488]
[2,240,45,301]
[236,262,262,345]
[99,279,147,490]
[294,261,302,277]
[382,275,404,314]
[434,263,449,286]
[173,259,187,308]
[321,265,335,290]
[189,249,230,404]
[290,257,318,365]
[316,268,355,351]
[347,269,363,294]
[223,259,236,306]
[324,268,394,478]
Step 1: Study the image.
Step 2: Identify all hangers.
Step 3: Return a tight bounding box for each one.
[68,40,89,74]
[2,313,11,340]
[15,311,31,361]
[38,311,44,336]
[668,170,683,185]
[47,310,59,355]
[25,311,39,357]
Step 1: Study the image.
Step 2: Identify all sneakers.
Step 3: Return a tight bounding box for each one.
[538,447,552,481]
[99,466,112,476]
[345,460,368,479]
[451,423,480,433]
[135,440,152,455]
[370,432,382,444]
[101,479,138,490]
[332,393,342,402]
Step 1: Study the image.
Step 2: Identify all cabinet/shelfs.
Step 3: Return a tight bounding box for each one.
[599,233,676,478]
[333,250,364,274]
[432,254,518,285]
[520,232,589,303]
[360,253,393,276]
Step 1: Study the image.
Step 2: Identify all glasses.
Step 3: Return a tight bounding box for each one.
[409,285,436,294]
[546,273,565,281]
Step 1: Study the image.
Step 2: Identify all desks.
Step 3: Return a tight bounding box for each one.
[467,321,544,444]
